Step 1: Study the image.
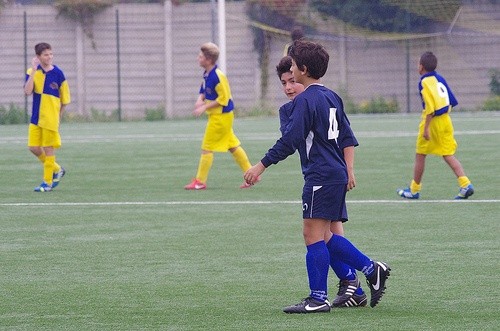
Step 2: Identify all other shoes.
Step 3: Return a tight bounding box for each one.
[241,174,260,187]
[185,177,207,189]
[52,166,66,187]
[34,182,53,192]
[395,188,419,199]
[454,184,474,200]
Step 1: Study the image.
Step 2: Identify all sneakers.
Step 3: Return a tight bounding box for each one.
[332,272,360,305]
[339,291,367,307]
[366,261,391,308]
[283,296,331,313]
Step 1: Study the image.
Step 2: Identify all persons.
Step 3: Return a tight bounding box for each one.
[184,43,261,190]
[243,39,391,313]
[277,56,367,307]
[284,29,304,57]
[24,43,71,193]
[396,51,475,200]
